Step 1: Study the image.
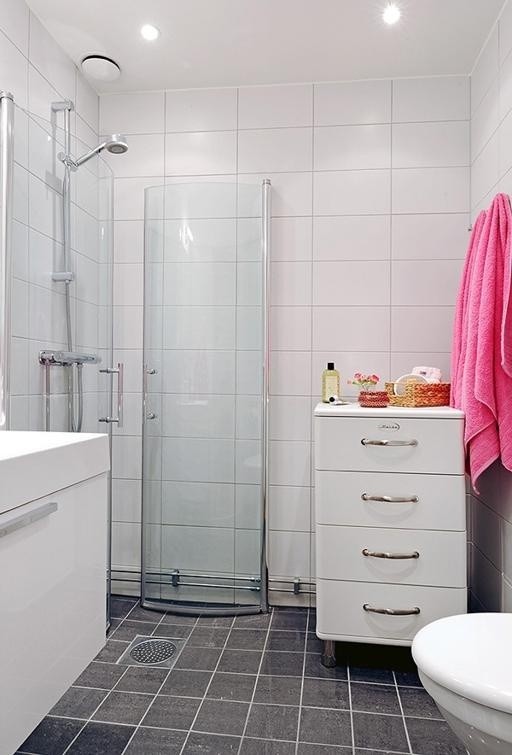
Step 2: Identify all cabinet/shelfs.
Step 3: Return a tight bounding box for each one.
[1,469,108,743]
[311,401,468,665]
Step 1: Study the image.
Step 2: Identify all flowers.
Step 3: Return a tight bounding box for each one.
[346,371,380,393]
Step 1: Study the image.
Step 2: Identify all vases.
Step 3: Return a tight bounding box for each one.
[359,389,388,408]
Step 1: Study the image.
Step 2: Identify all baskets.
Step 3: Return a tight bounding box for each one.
[385,381,450,408]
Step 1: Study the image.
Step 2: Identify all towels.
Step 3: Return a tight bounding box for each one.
[451,190,512,492]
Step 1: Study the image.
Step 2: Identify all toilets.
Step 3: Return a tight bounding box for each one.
[410,613,512,755]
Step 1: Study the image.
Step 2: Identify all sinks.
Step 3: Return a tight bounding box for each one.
[0,430,112,513]
